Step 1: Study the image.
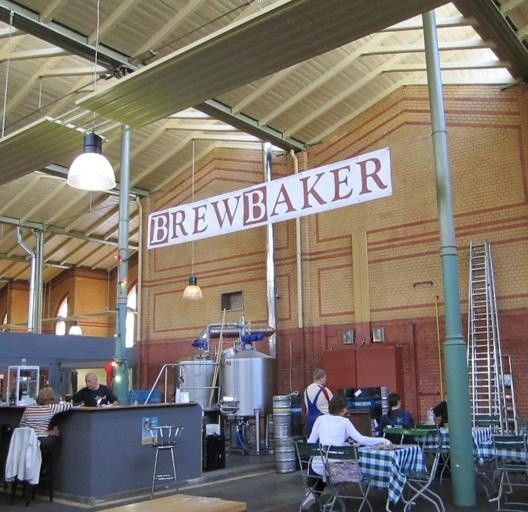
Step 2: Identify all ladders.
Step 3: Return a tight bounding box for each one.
[499,353,518,437]
[474,239,509,437]
[468,239,504,437]
[466,257,498,435]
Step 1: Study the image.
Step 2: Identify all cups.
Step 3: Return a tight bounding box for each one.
[65,393,73,402]
[395,416,401,426]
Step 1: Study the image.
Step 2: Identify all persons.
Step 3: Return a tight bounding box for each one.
[304,368,333,445]
[4,387,73,488]
[66,372,119,406]
[379,393,417,446]
[299,395,392,512]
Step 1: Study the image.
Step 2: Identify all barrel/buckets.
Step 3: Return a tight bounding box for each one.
[380,386,389,414]
[272,395,297,474]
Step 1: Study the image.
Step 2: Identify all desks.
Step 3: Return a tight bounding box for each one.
[315,444,428,511]
[95,494,248,511]
[479,439,528,466]
[409,427,492,452]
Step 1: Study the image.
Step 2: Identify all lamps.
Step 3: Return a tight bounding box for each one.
[68,321,83,336]
[183,140,204,300]
[67,0,116,192]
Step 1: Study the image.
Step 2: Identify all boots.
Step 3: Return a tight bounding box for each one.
[299,488,318,512]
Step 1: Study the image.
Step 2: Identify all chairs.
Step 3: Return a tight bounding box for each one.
[416,424,439,429]
[148,425,184,498]
[383,428,404,445]
[294,440,345,511]
[323,444,373,511]
[467,439,497,500]
[493,436,528,512]
[1,423,26,496]
[4,428,53,507]
[385,433,446,512]
[399,429,428,448]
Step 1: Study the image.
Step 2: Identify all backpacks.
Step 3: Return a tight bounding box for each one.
[384,410,413,440]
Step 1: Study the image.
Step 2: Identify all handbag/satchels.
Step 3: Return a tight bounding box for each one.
[326,461,363,487]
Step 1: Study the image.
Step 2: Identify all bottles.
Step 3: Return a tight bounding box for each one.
[21,358,27,365]
[9,388,16,406]
